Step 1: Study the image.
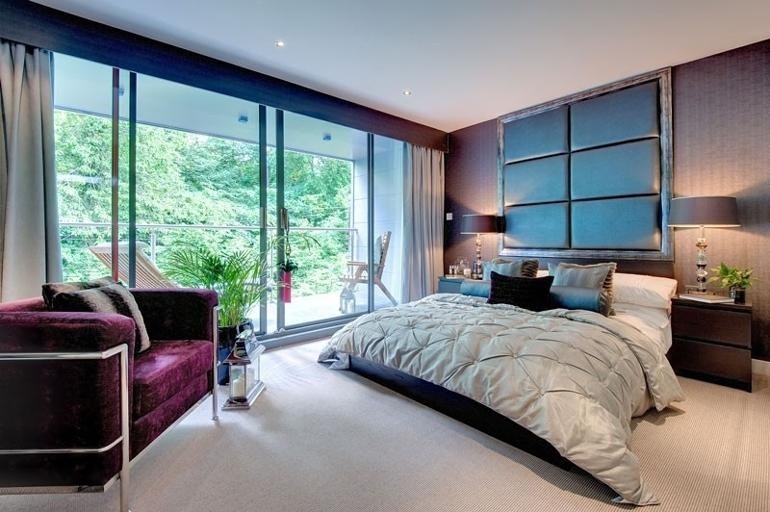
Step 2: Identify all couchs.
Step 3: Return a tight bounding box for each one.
[0,288,219,512]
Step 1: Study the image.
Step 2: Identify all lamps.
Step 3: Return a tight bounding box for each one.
[459,215,499,280]
[667,195,741,296]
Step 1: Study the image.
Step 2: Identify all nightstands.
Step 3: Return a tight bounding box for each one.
[437,276,465,293]
[437,275,482,296]
[670,297,752,393]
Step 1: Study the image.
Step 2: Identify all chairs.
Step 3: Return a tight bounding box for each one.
[338,231,398,314]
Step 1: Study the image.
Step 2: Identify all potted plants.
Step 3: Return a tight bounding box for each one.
[162,234,320,385]
[706,261,765,305]
[276,254,298,303]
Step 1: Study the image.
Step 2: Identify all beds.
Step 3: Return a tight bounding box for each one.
[317,257,687,500]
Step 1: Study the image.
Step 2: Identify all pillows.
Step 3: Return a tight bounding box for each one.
[486,271,554,312]
[41,276,115,302]
[482,260,522,280]
[551,263,616,317]
[52,283,151,355]
[492,258,539,278]
[460,280,609,312]
